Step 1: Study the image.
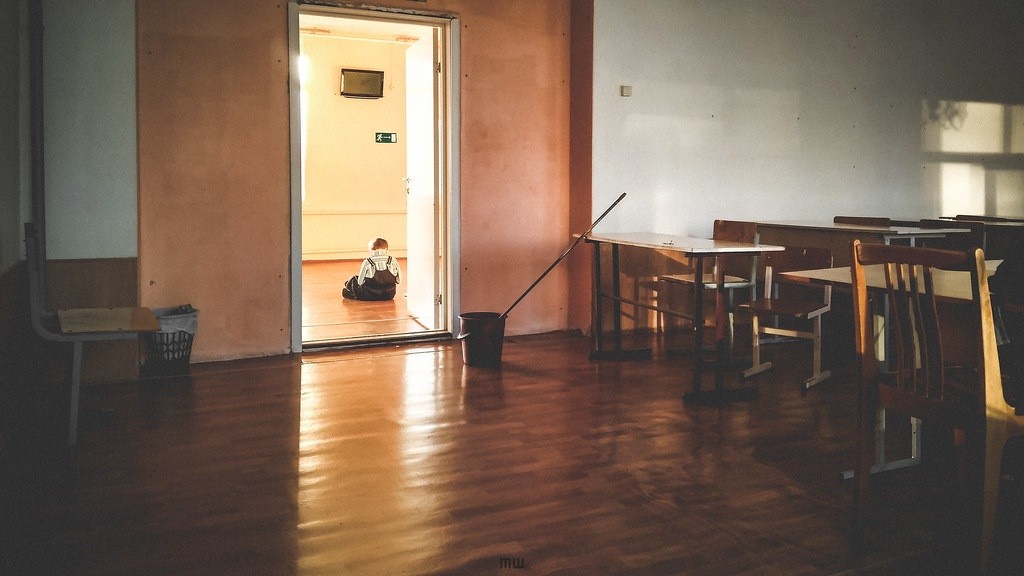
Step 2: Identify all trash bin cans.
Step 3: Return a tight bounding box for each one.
[144,303,200,374]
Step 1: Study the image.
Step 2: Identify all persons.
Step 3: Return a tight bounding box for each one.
[342,238,400,301]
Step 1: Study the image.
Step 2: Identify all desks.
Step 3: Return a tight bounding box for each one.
[572,233,787,406]
[939,216,1024,223]
[890,218,1024,254]
[778,260,1004,480]
[713,220,971,362]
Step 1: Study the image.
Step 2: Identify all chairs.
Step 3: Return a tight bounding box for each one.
[24,222,161,445]
[660,214,1024,576]
